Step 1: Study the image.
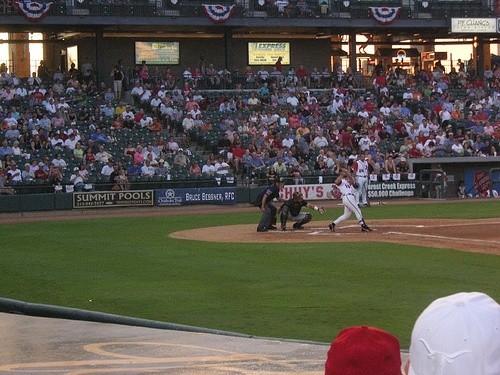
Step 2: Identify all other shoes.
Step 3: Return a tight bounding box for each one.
[281,227,286,231]
[257,228,268,231]
[268,225,276,229]
[293,223,304,229]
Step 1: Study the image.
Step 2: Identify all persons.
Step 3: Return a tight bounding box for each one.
[0,0,500,198]
[329,168,374,231]
[277,191,325,231]
[353,153,368,208]
[325,325,402,375]
[404,291,500,375]
[254,177,289,232]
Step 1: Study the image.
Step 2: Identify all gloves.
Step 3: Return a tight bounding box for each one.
[319,207,325,214]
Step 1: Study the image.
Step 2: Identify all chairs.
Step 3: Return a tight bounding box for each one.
[0,72,500,194]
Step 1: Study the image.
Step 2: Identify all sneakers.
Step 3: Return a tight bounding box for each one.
[328,224,335,232]
[362,226,372,232]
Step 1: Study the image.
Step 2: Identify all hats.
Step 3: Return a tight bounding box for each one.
[275,177,285,182]
[406,292,500,375]
[325,327,402,375]
